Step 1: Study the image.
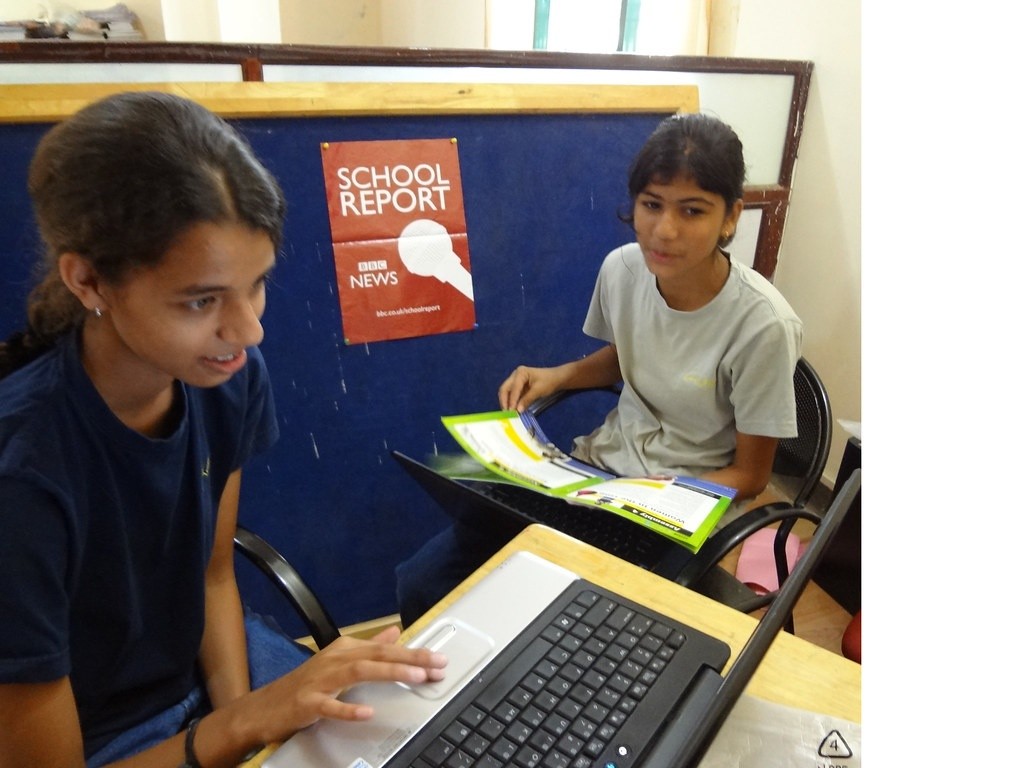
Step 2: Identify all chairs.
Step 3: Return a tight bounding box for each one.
[653,346,829,628]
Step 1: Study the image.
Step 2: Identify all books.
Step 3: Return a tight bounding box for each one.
[431,408,739,556]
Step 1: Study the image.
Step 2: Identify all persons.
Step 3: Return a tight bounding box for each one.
[499,113,806,540]
[3,92,447,768]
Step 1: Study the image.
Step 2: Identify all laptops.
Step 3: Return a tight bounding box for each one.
[257,468,861,768]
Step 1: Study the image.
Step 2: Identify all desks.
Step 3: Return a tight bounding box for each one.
[243,520,862,768]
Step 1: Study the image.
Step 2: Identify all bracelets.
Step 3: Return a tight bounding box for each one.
[184,716,204,767]
